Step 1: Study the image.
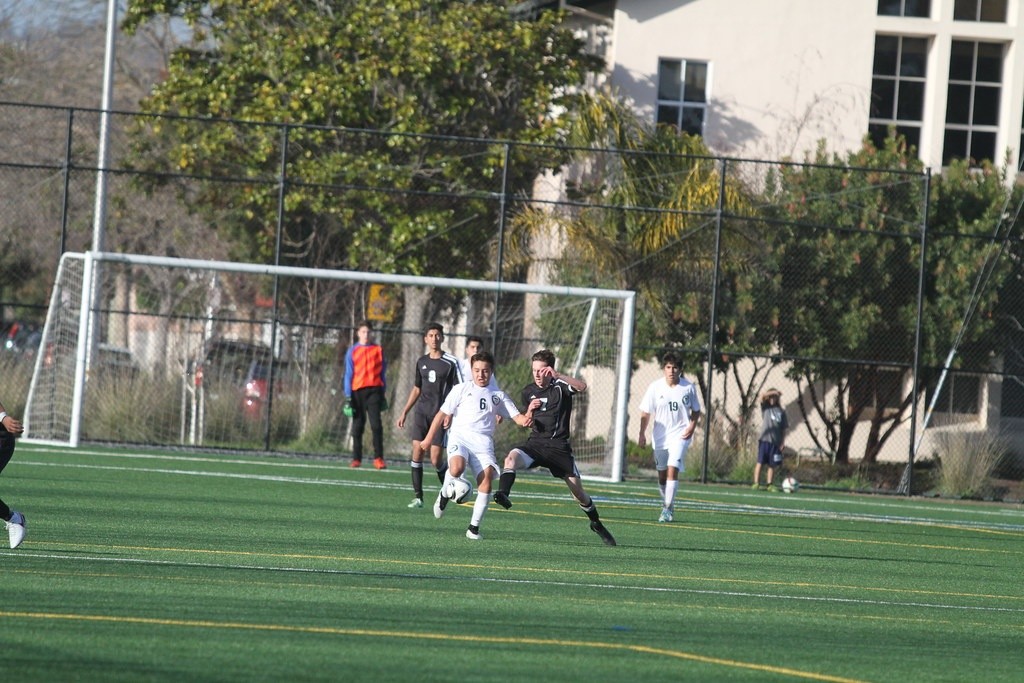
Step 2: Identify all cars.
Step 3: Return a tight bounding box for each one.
[0,316,144,394]
[179,333,352,445]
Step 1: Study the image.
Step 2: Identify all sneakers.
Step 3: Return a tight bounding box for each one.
[659,505,675,522]
[408,498,423,508]
[433,489,449,518]
[3,512,26,549]
[590,522,616,545]
[493,491,512,509]
[374,457,385,469]
[766,484,779,493]
[351,460,360,467]
[466,530,483,540]
[752,483,760,489]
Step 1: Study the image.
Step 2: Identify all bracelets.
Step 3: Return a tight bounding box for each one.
[0,412,7,422]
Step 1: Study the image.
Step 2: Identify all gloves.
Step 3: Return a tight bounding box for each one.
[381,399,388,411]
[343,398,356,417]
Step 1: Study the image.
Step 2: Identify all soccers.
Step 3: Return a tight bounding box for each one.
[782,477,799,495]
[446,478,474,504]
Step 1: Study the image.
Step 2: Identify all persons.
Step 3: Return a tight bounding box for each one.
[493,349,618,547]
[342,317,389,470]
[750,388,787,491]
[639,350,700,522]
[397,323,466,510]
[0,403,26,549]
[457,336,504,426]
[420,353,535,540]
